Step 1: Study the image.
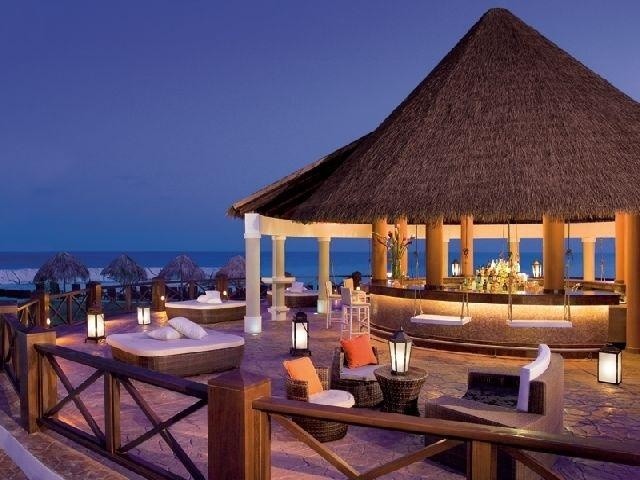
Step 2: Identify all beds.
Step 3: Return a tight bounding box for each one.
[165,300,246,324]
[266,289,318,309]
[106,327,244,377]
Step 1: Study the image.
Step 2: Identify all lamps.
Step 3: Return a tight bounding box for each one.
[83,301,107,343]
[135,296,151,324]
[288,309,311,356]
[451,259,462,277]
[597,341,622,385]
[388,324,413,375]
[531,259,543,278]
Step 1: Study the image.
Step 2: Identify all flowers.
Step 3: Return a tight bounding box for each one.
[371,224,416,278]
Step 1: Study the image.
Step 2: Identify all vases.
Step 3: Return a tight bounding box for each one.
[391,263,401,287]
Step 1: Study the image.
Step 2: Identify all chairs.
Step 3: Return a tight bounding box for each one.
[284,347,385,444]
[424,343,564,480]
[325,277,371,340]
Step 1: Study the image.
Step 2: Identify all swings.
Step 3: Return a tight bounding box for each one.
[410,216,472,326]
[506,218,573,328]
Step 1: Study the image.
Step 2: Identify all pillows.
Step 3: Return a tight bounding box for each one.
[282,355,323,397]
[287,281,308,293]
[196,290,222,303]
[339,333,377,368]
[144,316,208,339]
